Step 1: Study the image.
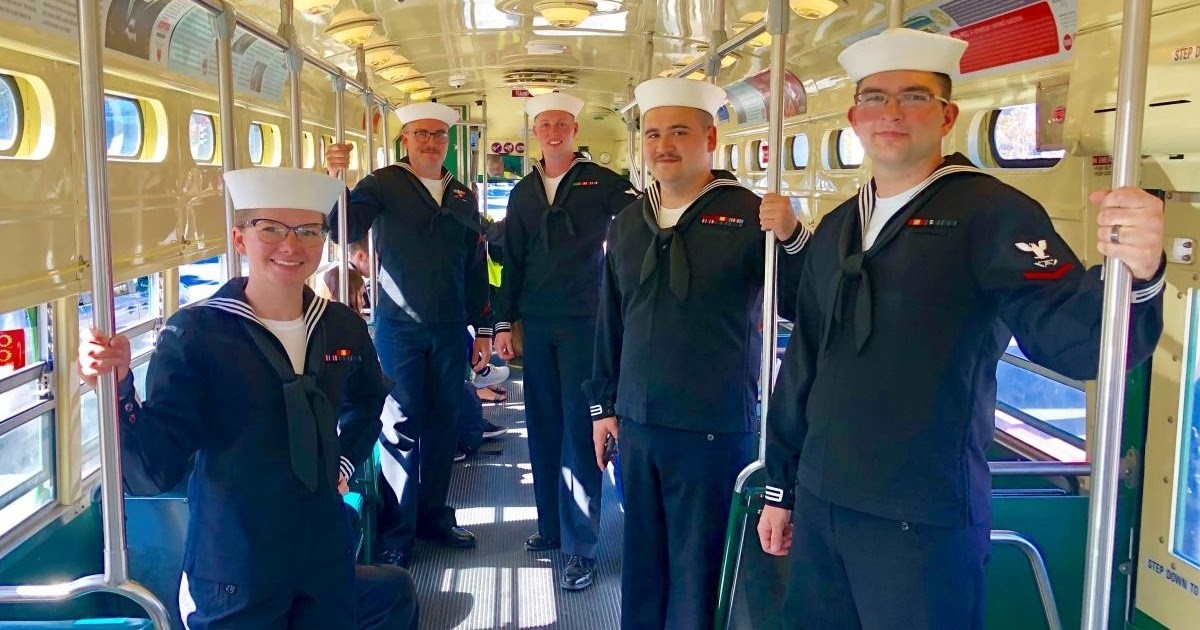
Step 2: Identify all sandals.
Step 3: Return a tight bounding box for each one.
[478,383,507,403]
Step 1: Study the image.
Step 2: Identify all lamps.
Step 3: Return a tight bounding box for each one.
[293,0,438,103]
[672,0,849,81]
[532,0,597,28]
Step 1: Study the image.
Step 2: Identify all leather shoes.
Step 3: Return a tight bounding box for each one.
[375,552,409,568]
[418,520,477,546]
[523,529,556,551]
[560,551,596,590]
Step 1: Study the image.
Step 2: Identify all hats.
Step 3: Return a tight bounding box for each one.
[222,167,346,216]
[525,92,584,120]
[635,77,728,124]
[395,101,460,128]
[836,25,968,85]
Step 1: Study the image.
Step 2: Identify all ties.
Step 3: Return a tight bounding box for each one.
[639,186,724,302]
[242,310,342,507]
[404,166,467,238]
[817,176,949,362]
[533,163,577,249]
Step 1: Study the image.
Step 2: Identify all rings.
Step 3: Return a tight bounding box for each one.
[1110,224,1123,245]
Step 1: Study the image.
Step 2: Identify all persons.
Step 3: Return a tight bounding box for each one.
[495,92,640,588]
[582,76,814,630]
[756,26,1168,630]
[77,101,508,630]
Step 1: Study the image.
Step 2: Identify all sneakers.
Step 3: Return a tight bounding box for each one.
[480,418,508,437]
[453,448,466,461]
[472,363,510,388]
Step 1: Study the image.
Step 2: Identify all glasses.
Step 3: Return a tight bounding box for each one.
[854,88,950,111]
[234,218,330,250]
[406,128,450,144]
